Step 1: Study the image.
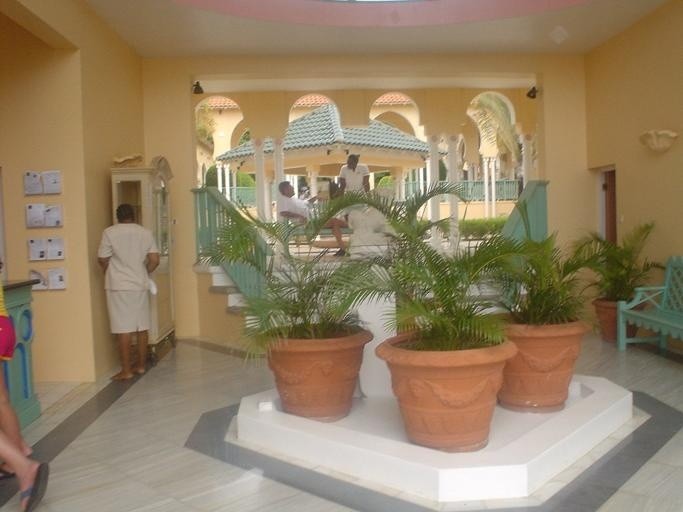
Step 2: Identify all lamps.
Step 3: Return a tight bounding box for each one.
[526,86,538,101]
[192,80,202,94]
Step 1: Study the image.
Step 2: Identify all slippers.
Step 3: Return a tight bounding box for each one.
[20,462,50,512]
[0,462,17,480]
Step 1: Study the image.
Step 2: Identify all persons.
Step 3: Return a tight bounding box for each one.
[0,286,48,512]
[96,203,160,381]
[277,180,347,250]
[333,154,371,256]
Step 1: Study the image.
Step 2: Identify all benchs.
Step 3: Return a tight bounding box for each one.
[616,253,682,356]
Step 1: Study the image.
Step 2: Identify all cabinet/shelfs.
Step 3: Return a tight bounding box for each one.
[110,154,174,364]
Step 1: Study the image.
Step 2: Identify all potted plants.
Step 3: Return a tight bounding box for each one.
[190,181,587,455]
[573,221,666,344]
